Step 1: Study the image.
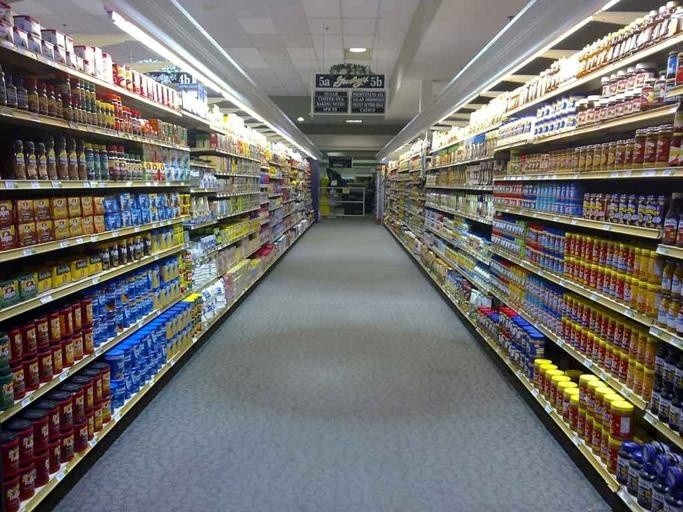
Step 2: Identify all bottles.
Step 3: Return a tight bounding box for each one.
[580,192,659,231]
[608,0,683,63]
[10,133,108,181]
[513,35,609,110]
[657,191,682,333]
[1,64,67,120]
[650,344,682,438]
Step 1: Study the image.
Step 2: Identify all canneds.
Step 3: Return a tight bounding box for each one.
[0,297,114,512]
[471,50,683,512]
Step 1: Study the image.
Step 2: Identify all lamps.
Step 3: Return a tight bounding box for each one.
[374,0,620,164]
[98,0,325,161]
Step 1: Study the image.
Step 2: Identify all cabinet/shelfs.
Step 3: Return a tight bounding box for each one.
[0,38,314,512]
[382,32,683,512]
[320,182,366,217]
